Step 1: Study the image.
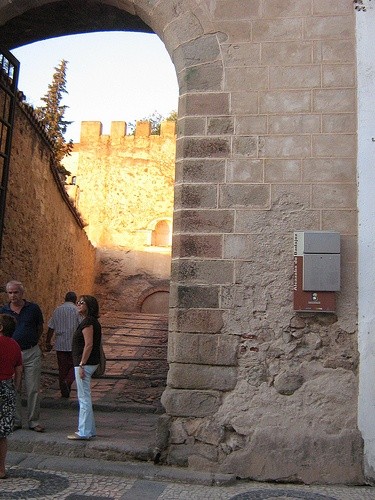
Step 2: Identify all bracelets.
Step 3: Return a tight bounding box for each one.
[79,361,85,369]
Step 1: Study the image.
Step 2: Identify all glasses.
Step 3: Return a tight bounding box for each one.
[77,301,87,304]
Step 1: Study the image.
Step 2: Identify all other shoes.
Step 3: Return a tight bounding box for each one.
[29,425,46,431]
[62,381,71,393]
[67,432,96,439]
[13,426,22,431]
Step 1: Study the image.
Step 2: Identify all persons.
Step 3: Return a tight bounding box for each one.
[0,281,47,433]
[67,295,102,441]
[0,314,23,479]
[45,292,86,399]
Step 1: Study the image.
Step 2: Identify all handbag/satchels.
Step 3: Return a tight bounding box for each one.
[91,344,106,378]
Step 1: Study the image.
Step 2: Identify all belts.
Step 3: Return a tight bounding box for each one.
[20,343,37,351]
